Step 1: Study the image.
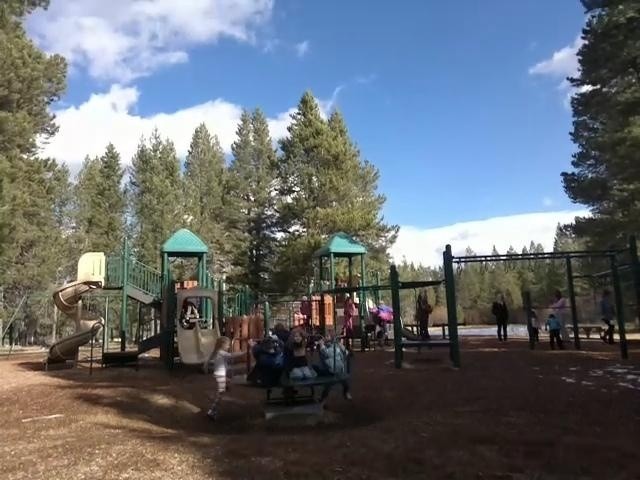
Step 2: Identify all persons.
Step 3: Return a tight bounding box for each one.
[492,293,508,341]
[252,323,352,399]
[208,336,247,421]
[531,311,541,342]
[545,290,569,350]
[418,289,429,336]
[344,297,355,330]
[600,290,615,345]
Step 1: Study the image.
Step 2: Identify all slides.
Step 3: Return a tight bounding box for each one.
[45,280,105,365]
[354,297,419,341]
[176,319,221,366]
